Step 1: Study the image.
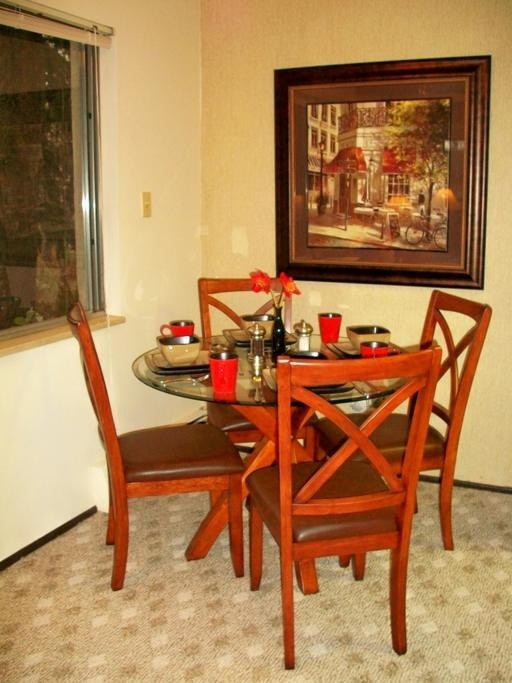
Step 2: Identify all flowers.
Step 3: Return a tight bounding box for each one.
[250,269,302,307]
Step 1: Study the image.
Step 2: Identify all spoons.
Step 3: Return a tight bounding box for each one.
[249,375,263,401]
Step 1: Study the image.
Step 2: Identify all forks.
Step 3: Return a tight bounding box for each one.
[156,372,210,385]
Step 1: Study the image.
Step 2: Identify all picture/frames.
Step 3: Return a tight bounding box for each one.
[274,55,491,291]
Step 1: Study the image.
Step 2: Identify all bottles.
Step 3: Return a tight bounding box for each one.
[246,323,267,378]
[294,319,312,351]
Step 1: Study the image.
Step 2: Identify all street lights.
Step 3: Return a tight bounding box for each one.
[316,141,326,214]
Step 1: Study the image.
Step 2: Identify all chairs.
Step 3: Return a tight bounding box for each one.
[198,278,320,457]
[66,300,246,592]
[244,344,443,672]
[313,290,493,568]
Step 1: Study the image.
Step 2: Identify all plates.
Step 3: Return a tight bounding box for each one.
[326,341,401,356]
[262,368,355,395]
[222,327,298,347]
[146,348,213,373]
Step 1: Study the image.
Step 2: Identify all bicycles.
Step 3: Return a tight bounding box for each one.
[404,210,447,247]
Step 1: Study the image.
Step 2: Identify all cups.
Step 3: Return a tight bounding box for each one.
[318,311,342,342]
[160,321,195,338]
[359,340,390,359]
[212,391,237,403]
[208,350,240,393]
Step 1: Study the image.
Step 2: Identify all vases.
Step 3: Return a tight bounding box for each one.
[271,306,285,363]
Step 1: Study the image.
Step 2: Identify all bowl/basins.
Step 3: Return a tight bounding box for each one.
[157,334,202,365]
[346,324,391,348]
[240,313,276,336]
[285,348,329,359]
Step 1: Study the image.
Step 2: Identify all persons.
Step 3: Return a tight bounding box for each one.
[418,189,426,217]
